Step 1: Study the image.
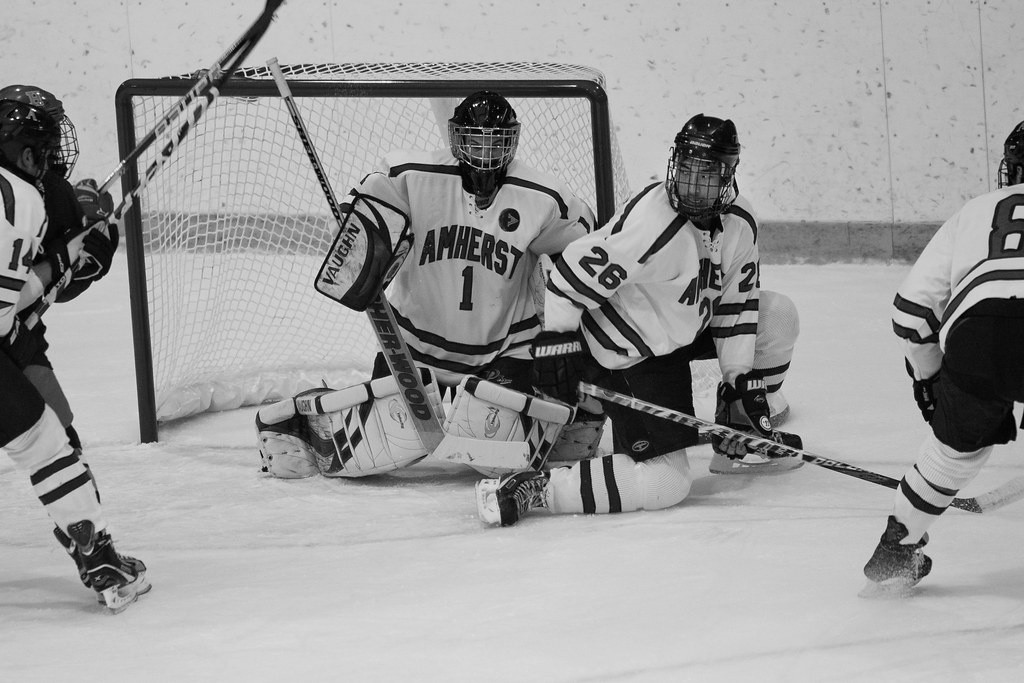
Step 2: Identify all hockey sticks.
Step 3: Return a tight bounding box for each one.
[9,1,284,345]
[266,56,530,465]
[575,378,1023,513]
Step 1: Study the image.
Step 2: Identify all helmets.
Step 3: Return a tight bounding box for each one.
[677,115,741,168]
[449,91,518,138]
[1003,120,1024,164]
[0,85,65,143]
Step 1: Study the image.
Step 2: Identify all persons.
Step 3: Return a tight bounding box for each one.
[477,113,809,529]
[1,83,156,615]
[259,91,613,476]
[861,121,1024,588]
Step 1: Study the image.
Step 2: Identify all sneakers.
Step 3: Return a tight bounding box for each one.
[858,514,933,599]
[55,530,108,605]
[708,371,806,474]
[70,521,152,615]
[473,469,550,527]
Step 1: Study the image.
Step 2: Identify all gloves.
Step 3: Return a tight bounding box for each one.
[73,226,121,292]
[66,178,113,238]
